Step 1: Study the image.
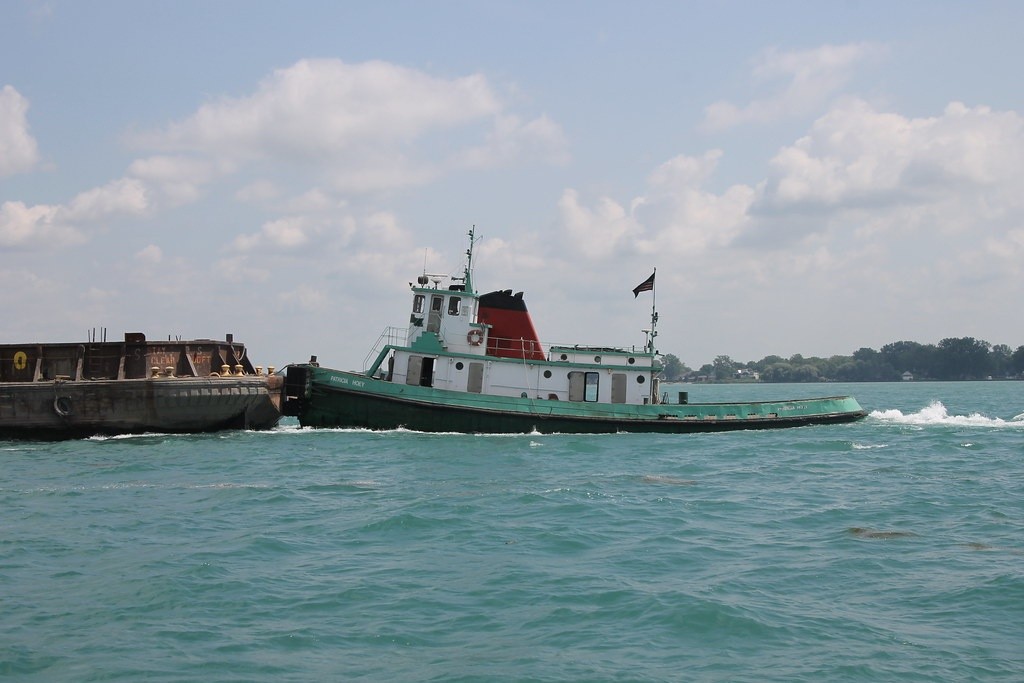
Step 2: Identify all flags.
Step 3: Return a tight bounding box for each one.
[633,273,654,298]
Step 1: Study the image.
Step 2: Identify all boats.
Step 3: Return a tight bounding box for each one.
[281,225,868,434]
[0,333,285,440]
